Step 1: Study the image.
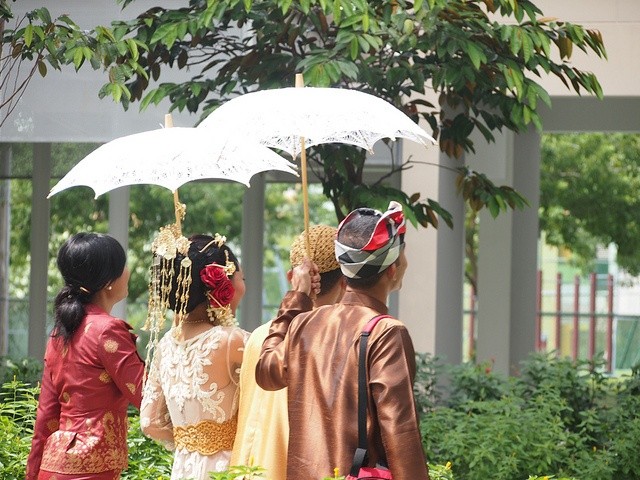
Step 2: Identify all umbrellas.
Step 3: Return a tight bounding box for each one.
[46,113,301,328]
[194,74,438,304]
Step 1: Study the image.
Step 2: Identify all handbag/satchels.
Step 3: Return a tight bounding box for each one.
[345,315,399,480]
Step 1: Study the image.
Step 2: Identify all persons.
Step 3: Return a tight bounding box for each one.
[230,223,346,480]
[26,232,148,480]
[140,203,252,480]
[255,201,430,480]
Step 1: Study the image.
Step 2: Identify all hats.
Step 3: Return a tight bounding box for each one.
[290,225,340,274]
